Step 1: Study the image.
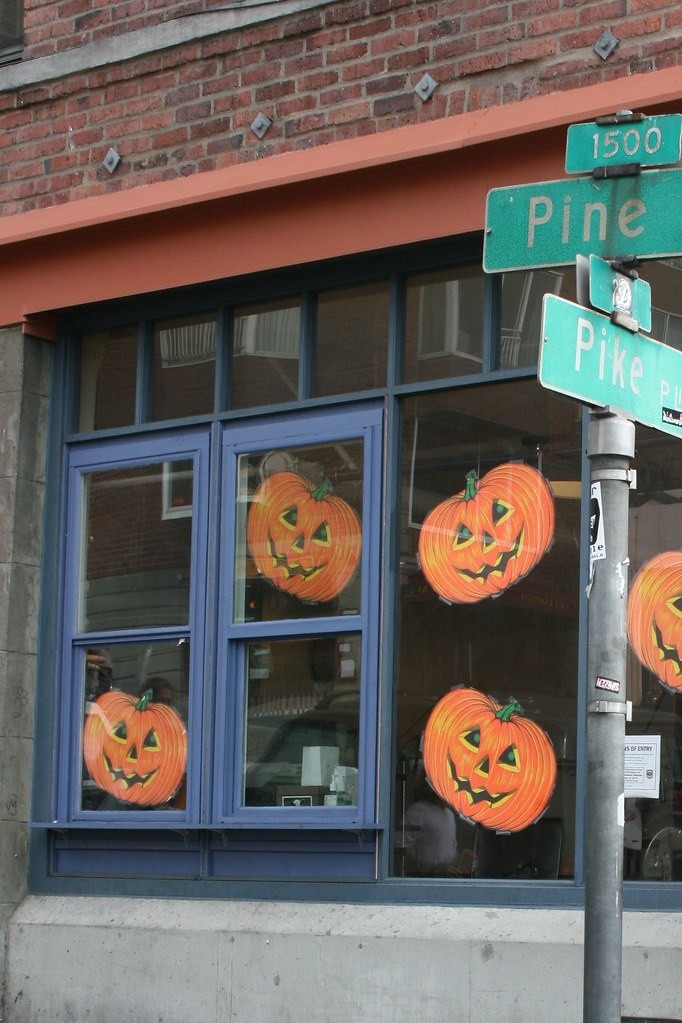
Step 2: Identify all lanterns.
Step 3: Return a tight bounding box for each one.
[245,466,362,604]
[625,550,681,697]
[418,461,558,607]
[418,686,558,834]
[84,682,187,808]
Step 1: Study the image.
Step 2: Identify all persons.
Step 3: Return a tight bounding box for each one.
[139,675,175,709]
[400,786,460,874]
